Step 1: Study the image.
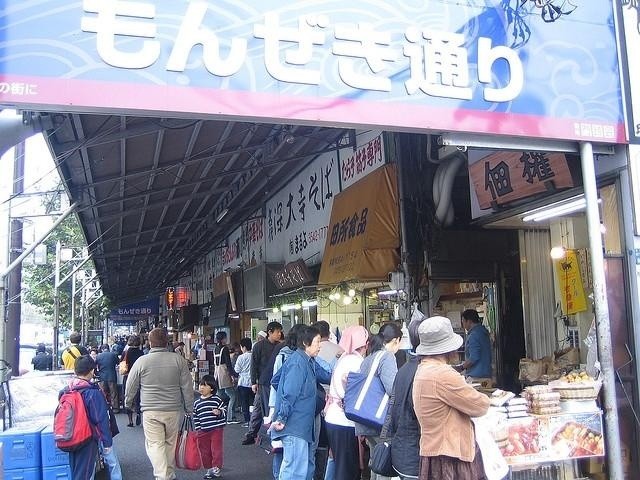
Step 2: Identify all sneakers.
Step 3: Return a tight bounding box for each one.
[240,435,255,445]
[203,466,223,479]
[226,418,249,428]
[127,415,142,428]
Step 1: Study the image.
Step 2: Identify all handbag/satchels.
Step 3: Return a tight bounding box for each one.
[368,444,399,477]
[216,347,234,390]
[94,458,109,480]
[118,361,129,376]
[255,423,282,454]
[344,367,391,428]
[174,416,202,470]
[315,383,327,417]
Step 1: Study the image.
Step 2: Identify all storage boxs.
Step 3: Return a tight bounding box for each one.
[0,468,41,480]
[41,465,72,480]
[1,423,48,466]
[42,424,69,467]
[580,416,634,474]
[591,472,634,480]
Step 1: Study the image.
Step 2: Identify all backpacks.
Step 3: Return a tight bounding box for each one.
[51,380,100,453]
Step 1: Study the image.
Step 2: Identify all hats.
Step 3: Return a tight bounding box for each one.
[415,315,464,356]
[70,332,81,343]
[259,330,268,339]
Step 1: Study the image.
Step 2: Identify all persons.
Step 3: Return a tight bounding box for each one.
[322,324,369,479]
[353,320,403,479]
[388,315,429,479]
[411,315,492,480]
[458,309,493,388]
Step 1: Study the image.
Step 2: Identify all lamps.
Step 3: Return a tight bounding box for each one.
[280,124,297,146]
